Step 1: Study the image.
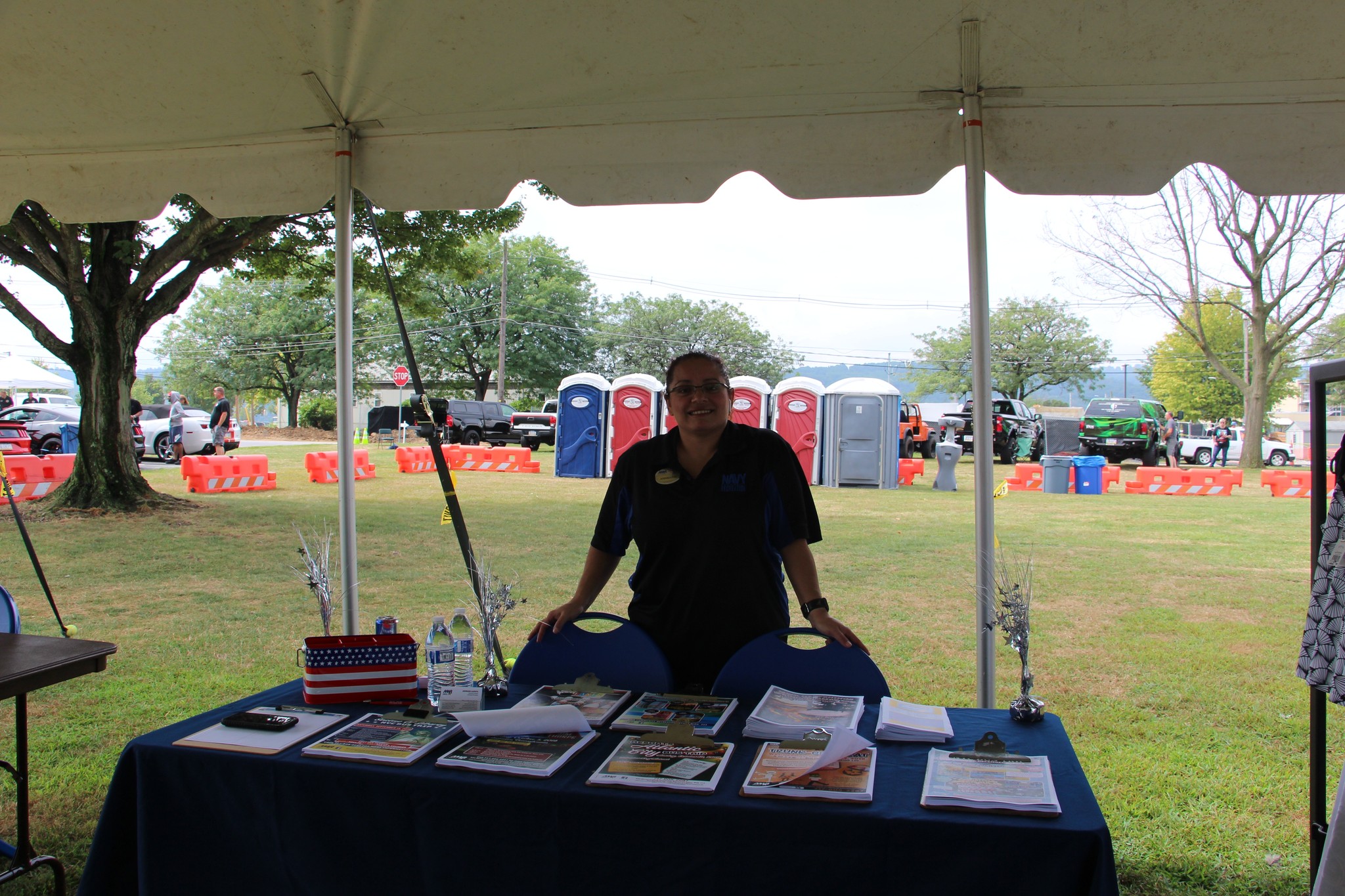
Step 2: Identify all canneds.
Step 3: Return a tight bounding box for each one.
[375,615,400,634]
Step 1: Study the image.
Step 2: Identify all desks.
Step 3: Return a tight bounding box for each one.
[81,674,1117,896]
[0,632,117,896]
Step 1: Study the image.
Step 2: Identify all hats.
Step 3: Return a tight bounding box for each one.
[168,392,171,396]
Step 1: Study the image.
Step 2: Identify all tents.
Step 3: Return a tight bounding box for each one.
[0,353,75,420]
[0,1,1345,706]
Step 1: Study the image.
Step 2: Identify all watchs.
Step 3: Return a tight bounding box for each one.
[800,597,829,620]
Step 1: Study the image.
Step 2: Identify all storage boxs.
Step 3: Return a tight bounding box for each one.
[297,633,420,704]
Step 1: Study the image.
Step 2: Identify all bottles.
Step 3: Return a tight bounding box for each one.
[447,608,474,687]
[425,616,456,706]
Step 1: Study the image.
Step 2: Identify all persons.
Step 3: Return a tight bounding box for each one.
[527,351,869,695]
[1231,422,1237,440]
[1156,412,1179,467]
[209,387,231,455]
[130,398,143,423]
[165,391,185,465]
[179,395,190,406]
[22,392,39,417]
[1207,418,1232,467]
[0,391,14,419]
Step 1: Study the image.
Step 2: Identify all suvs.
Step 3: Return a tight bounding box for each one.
[1078,397,1186,467]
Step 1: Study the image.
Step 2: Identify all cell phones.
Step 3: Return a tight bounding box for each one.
[221,712,299,731]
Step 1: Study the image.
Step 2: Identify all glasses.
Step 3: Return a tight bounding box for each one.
[214,390,221,392]
[0,395,4,397]
[665,382,730,398]
[168,396,170,397]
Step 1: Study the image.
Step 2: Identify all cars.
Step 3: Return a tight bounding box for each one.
[0,419,32,456]
[899,400,937,460]
[12,393,78,406]
[0,403,146,464]
[132,404,242,463]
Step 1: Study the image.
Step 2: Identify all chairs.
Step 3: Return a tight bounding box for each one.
[39,424,80,455]
[710,628,891,703]
[378,429,395,450]
[439,423,450,445]
[509,613,673,694]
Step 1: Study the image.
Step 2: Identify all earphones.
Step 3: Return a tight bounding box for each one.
[729,399,732,413]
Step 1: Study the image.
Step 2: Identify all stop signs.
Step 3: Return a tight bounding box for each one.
[392,366,408,387]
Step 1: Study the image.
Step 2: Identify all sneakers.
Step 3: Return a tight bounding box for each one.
[174,460,181,464]
[165,458,178,464]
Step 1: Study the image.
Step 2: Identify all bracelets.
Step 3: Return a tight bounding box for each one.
[133,415,136,418]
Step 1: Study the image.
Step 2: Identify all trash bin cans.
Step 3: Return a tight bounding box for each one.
[1040,455,1073,493]
[1072,455,1105,494]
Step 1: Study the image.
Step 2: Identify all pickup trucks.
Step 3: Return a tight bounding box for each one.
[1174,422,1296,468]
[510,399,558,451]
[941,398,1046,467]
[412,398,525,448]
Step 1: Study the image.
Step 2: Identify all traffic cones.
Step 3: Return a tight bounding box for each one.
[352,427,363,446]
[361,429,369,444]
